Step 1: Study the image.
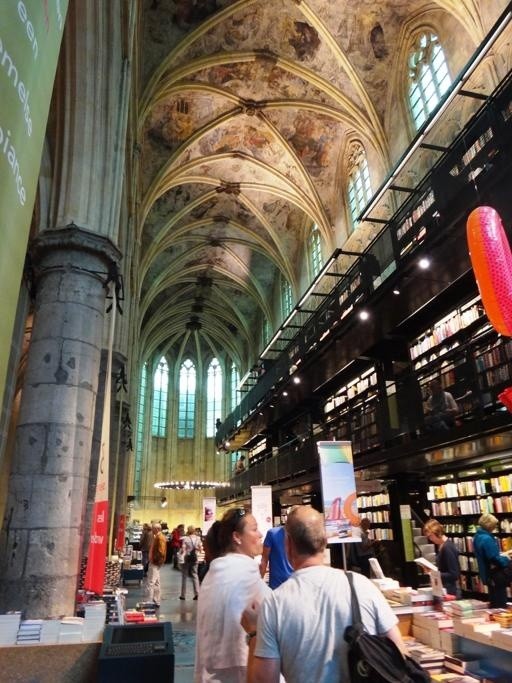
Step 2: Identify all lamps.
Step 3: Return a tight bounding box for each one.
[153,480,230,491]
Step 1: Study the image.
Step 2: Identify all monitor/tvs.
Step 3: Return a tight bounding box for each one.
[111,627,164,644]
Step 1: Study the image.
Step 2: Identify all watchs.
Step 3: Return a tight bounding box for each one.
[245,632,256,644]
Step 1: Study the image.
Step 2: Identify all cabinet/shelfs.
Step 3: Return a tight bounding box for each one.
[249,438,267,468]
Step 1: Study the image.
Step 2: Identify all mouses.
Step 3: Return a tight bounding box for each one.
[155,643,165,652]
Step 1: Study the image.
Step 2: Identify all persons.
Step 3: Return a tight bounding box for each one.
[420,516,462,597]
[424,381,460,430]
[234,455,245,474]
[240,504,422,681]
[137,517,201,611]
[195,507,275,683]
[356,516,380,576]
[216,418,222,428]
[258,524,291,592]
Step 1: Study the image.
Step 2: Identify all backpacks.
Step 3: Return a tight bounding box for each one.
[341,570,430,683]
[186,535,199,566]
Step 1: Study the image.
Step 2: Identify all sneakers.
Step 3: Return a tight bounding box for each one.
[193,593,199,601]
[178,595,185,600]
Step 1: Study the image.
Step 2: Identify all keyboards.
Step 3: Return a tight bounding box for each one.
[105,644,153,655]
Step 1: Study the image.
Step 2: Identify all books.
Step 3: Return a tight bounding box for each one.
[448,126,497,195]
[427,473,512,600]
[324,368,383,455]
[357,492,393,543]
[0,597,108,648]
[288,279,359,374]
[76,520,157,626]
[280,503,290,524]
[365,557,511,682]
[395,186,433,256]
[410,298,510,425]
[248,437,267,465]
